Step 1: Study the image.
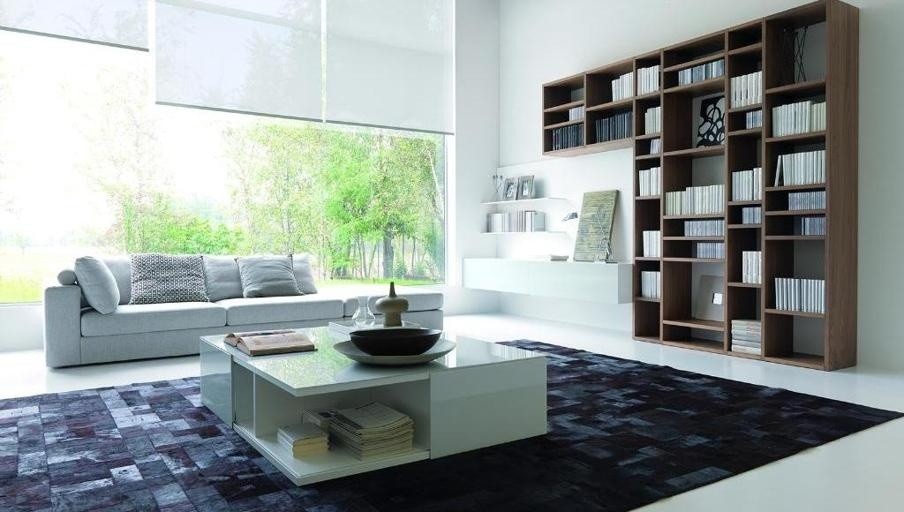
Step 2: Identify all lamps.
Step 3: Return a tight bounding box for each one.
[561,209,621,266]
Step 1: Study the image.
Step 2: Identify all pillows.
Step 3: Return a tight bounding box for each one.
[237,254,297,298]
[76,256,122,315]
[129,252,206,303]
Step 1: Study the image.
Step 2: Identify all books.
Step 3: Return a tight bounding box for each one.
[773,101,826,138]
[643,231,660,257]
[742,251,763,284]
[650,139,660,154]
[666,184,725,216]
[731,168,761,224]
[222,329,318,356]
[789,191,827,211]
[550,72,633,153]
[639,167,660,196]
[489,208,546,232]
[802,217,826,235]
[276,399,417,462]
[645,107,661,134]
[637,64,660,95]
[642,271,660,299]
[679,59,724,86]
[731,320,761,355]
[730,71,762,129]
[685,221,725,258]
[775,149,828,187]
[775,277,825,314]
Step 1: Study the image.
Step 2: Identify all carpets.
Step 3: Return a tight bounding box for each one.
[1,337,903,511]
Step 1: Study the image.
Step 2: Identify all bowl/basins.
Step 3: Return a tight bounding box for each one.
[350,328,440,355]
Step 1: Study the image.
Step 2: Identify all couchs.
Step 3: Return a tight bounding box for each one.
[42,248,463,370]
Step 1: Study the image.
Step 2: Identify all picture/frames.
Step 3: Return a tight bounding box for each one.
[502,175,519,200]
[519,175,534,199]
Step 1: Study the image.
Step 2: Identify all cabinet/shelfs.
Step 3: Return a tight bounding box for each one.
[462,196,633,305]
[539,0,861,370]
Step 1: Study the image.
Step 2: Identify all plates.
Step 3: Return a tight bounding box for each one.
[332,338,454,365]
